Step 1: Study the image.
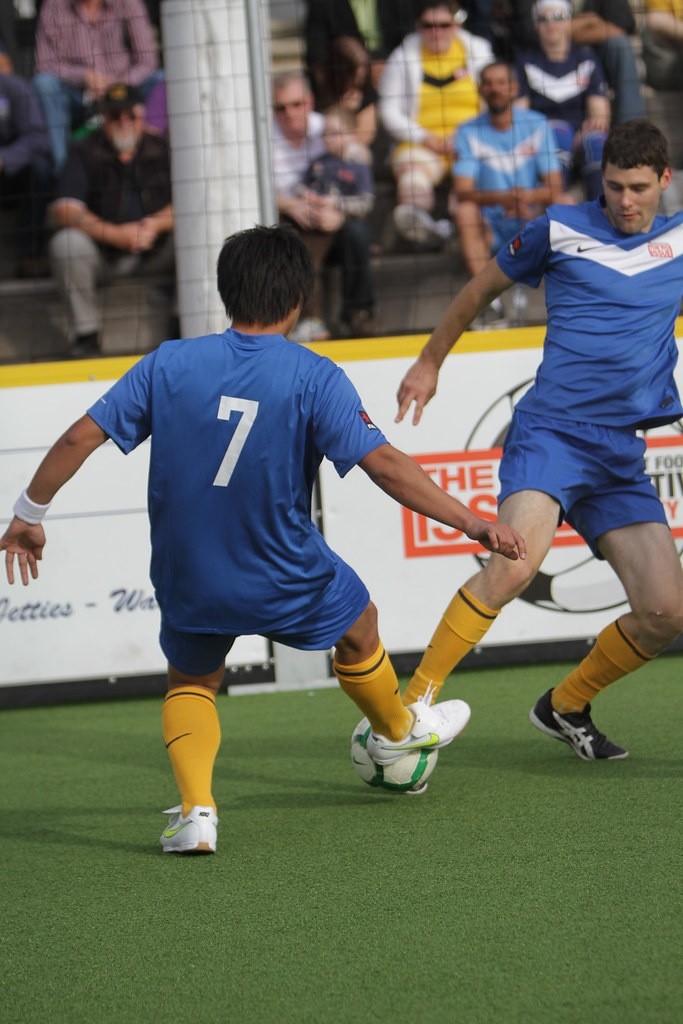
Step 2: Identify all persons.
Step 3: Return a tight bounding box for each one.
[270,0,683,337]
[1,0,182,359]
[0,224,527,851]
[396,123,683,761]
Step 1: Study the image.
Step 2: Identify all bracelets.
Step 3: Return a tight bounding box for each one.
[14,489,51,524]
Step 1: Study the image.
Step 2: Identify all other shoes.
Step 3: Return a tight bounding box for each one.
[167,318,180,340]
[464,290,524,330]
[339,312,378,338]
[286,318,329,344]
[65,334,101,360]
[394,206,453,251]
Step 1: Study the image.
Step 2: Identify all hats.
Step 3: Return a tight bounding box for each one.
[98,83,142,115]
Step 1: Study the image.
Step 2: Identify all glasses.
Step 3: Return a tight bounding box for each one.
[422,23,453,29]
[274,102,304,112]
[536,15,569,22]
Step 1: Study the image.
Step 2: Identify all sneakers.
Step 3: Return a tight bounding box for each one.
[366,679,471,766]
[160,805,218,851]
[529,687,629,761]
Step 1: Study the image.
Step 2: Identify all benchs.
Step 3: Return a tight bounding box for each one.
[0,168,476,296]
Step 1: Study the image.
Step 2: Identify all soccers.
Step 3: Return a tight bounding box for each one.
[350,715,439,794]
